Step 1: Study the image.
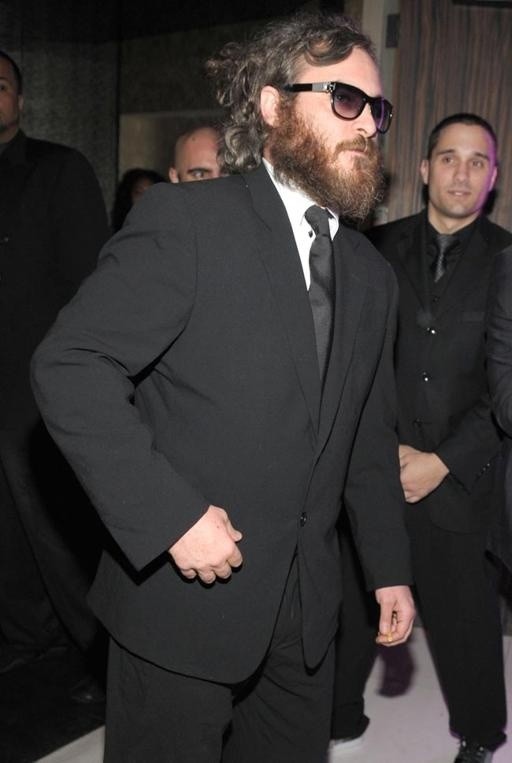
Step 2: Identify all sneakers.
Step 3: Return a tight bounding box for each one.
[378,654,415,698]
[66,669,107,706]
[453,736,495,763]
[0,639,45,675]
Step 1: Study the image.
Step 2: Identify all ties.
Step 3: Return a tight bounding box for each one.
[303,204,333,388]
[432,233,460,281]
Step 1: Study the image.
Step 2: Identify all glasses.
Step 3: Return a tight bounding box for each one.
[284,81,393,133]
